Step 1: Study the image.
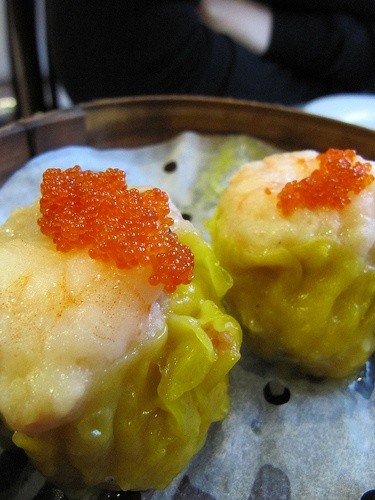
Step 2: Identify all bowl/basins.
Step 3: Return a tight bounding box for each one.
[0,95,375,190]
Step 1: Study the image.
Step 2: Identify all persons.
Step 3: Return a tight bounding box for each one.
[53,0,375,107]
